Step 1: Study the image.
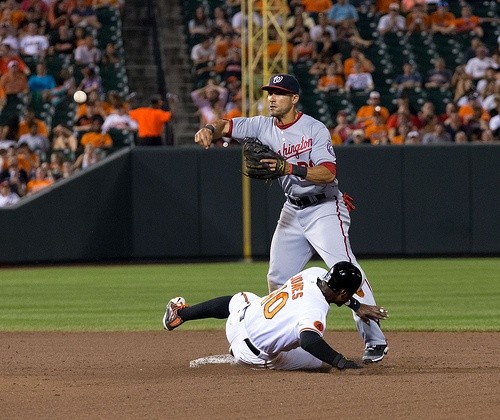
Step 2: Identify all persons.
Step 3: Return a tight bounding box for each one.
[162,261,390,372]
[188,0,500,145]
[0,0,175,208]
[195,75,389,362]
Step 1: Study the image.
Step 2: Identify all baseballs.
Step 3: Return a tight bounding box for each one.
[73,90,87,103]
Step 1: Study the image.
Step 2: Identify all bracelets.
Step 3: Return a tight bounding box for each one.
[203,125,214,134]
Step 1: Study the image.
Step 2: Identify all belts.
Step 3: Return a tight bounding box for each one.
[244,338,260,356]
[288,193,326,207]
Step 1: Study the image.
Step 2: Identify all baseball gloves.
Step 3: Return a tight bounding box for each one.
[243,141,289,181]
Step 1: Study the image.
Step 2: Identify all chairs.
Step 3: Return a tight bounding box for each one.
[1,8,127,124]
[296,0,500,123]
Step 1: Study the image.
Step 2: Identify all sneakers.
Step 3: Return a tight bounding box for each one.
[163,297,189,331]
[362,344,390,365]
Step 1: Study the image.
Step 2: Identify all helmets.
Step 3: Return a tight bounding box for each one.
[323,261,362,298]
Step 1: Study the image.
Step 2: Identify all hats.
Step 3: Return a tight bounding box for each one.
[390,3,399,10]
[260,75,300,94]
